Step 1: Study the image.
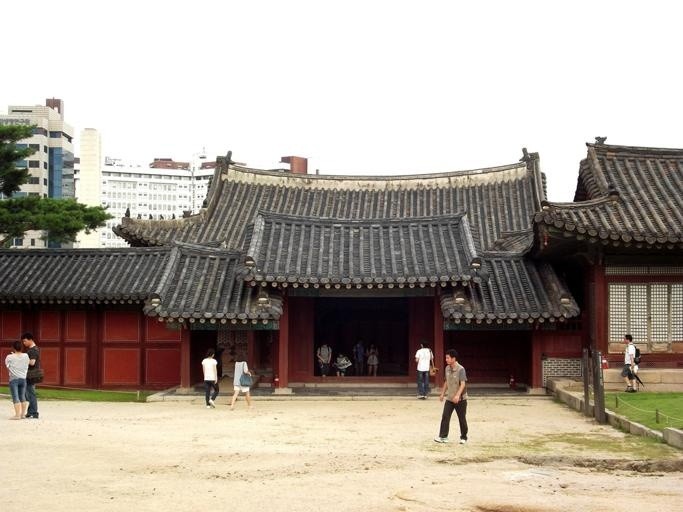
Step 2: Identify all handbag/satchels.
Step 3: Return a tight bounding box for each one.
[429,366,439,376]
[627,344,640,364]
[27,368,43,384]
[240,374,254,386]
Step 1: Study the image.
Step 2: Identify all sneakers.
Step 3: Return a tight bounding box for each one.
[417,395,427,399]
[460,439,466,444]
[209,398,216,407]
[434,437,448,443]
[10,414,38,420]
[626,385,637,393]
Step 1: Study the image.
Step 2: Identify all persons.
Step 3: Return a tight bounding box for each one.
[621,335,639,392]
[414,341,438,399]
[201,348,220,408]
[229,353,251,409]
[20,333,40,418]
[4,341,30,420]
[317,339,379,378]
[434,349,468,444]
